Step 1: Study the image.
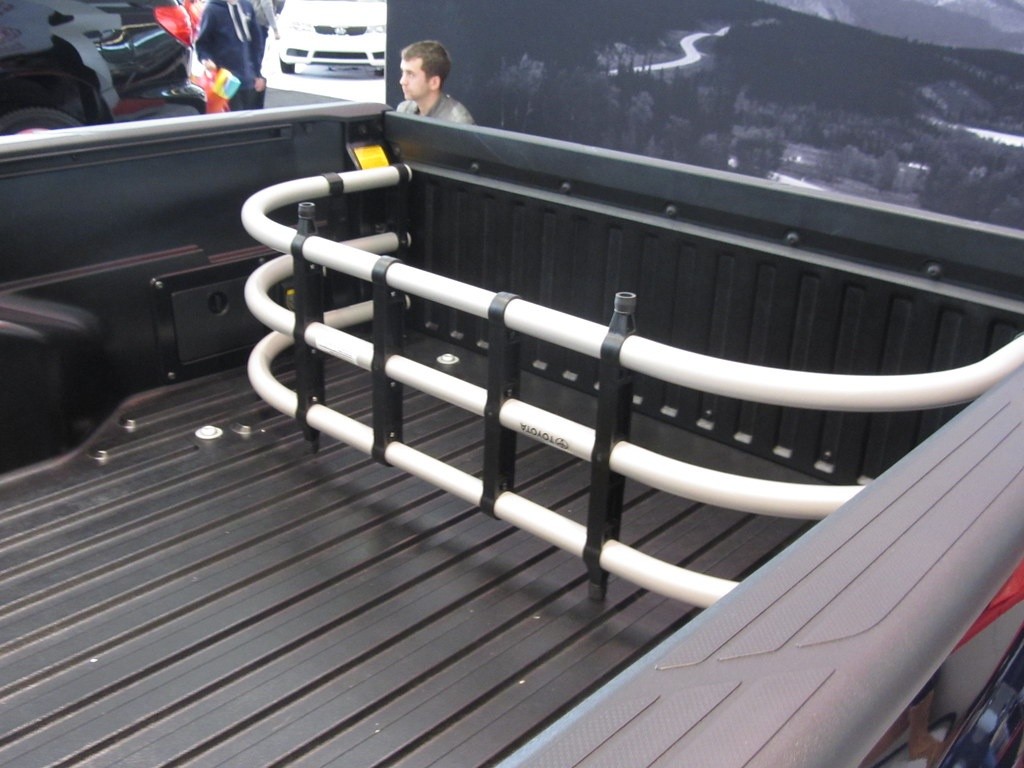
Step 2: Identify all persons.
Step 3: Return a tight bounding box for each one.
[397,40,474,124]
[178,0,285,111]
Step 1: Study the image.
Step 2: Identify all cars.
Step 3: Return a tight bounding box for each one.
[278,1,388,76]
